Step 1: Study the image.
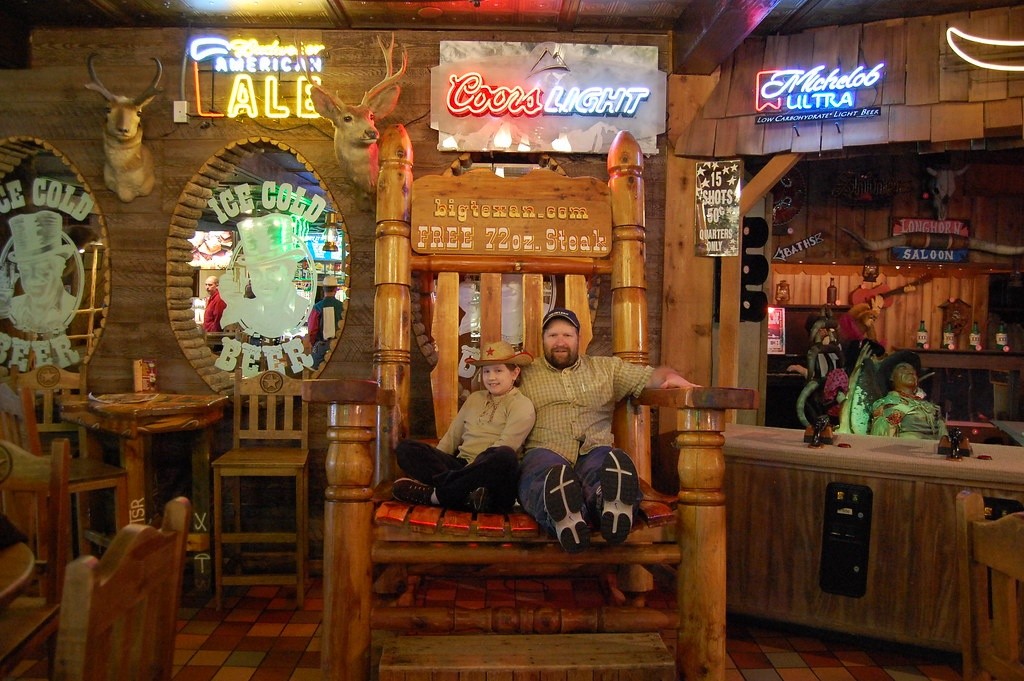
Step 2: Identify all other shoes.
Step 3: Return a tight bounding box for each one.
[542,465,591,554]
[596,448,638,545]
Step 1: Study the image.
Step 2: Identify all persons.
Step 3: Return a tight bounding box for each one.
[788,314,846,427]
[392,340,535,513]
[518,310,702,553]
[308,276,345,371]
[870,351,950,440]
[204,276,226,352]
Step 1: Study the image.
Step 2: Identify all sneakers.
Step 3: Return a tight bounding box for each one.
[453,486,490,513]
[391,478,441,508]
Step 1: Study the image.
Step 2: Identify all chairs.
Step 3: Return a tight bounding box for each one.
[953,488,1024,681]
[0,362,192,681]
[211,367,310,610]
[301,124,758,681]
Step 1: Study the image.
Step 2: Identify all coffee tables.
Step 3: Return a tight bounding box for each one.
[60,391,229,596]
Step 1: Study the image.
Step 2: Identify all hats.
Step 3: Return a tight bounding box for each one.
[542,307,580,330]
[465,340,534,366]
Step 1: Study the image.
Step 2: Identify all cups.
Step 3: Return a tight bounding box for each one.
[227,267,244,294]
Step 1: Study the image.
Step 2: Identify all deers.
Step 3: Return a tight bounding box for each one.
[85,52,162,202]
[294,31,406,213]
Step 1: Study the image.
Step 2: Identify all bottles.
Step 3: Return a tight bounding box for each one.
[943,322,956,349]
[968,321,980,350]
[994,322,1009,350]
[826,277,837,305]
[917,319,928,348]
